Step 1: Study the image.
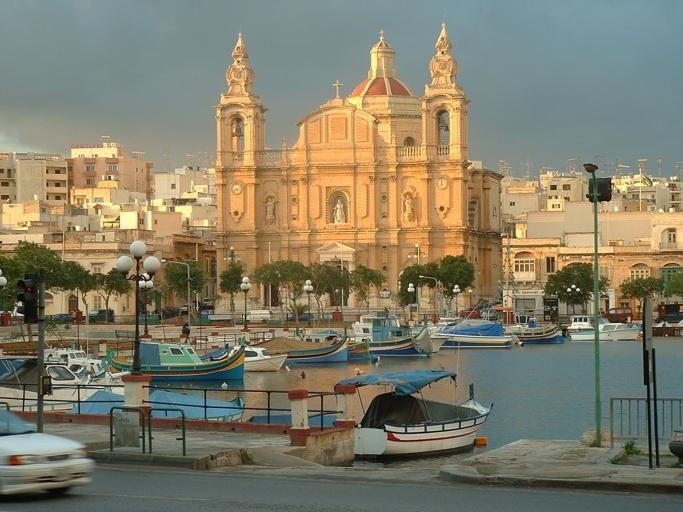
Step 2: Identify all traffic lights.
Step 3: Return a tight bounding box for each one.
[16,272,39,324]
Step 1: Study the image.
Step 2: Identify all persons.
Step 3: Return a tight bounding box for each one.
[182,324,190,335]
[331,199,346,223]
[405,195,413,213]
[267,197,274,218]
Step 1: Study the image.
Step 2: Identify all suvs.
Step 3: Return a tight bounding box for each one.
[87,308,115,323]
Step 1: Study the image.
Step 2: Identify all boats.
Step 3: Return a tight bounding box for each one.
[507,314,642,345]
[334,369,494,462]
[227,345,290,373]
[250,335,349,364]
[0,347,131,414]
[106,339,247,384]
[434,321,518,350]
[348,307,450,364]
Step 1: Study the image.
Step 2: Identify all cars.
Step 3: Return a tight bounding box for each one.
[0,408,98,500]
[179,297,214,314]
[139,310,161,324]
[653,310,683,323]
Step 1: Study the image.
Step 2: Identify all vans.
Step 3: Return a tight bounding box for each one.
[52,313,73,322]
[599,307,634,324]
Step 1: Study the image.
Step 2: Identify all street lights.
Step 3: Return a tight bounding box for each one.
[239,275,253,333]
[160,259,193,335]
[582,161,612,447]
[114,240,161,377]
[221,246,241,315]
[566,283,581,314]
[451,284,462,319]
[140,272,156,339]
[407,282,416,322]
[303,279,316,328]
